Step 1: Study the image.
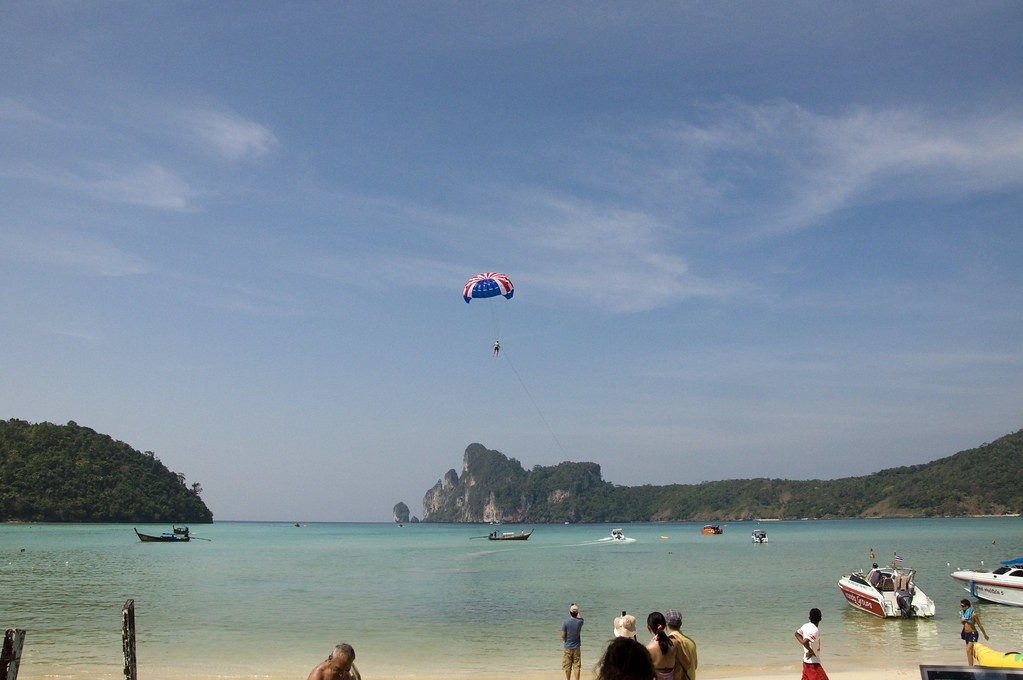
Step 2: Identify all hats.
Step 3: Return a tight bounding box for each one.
[570,603,579,613]
[664,608,683,626]
[873,563,878,568]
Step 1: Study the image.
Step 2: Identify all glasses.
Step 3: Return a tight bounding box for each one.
[961,604,966,608]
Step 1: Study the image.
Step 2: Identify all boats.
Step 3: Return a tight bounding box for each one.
[838,564,937,618]
[610,529,626,542]
[172,525,191,535]
[752,529,769,543]
[486,529,535,540]
[133,527,192,542]
[948,556,1023,607]
[700,524,724,535]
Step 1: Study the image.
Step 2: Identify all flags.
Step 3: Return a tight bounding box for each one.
[895,555,903,562]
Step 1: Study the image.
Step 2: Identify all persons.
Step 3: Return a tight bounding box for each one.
[960,599,989,666]
[870,549,874,560]
[795,608,829,680]
[599,615,654,680]
[645,612,679,680]
[560,605,584,680]
[867,563,882,585]
[307,643,361,680]
[491,340,500,357]
[665,609,698,680]
